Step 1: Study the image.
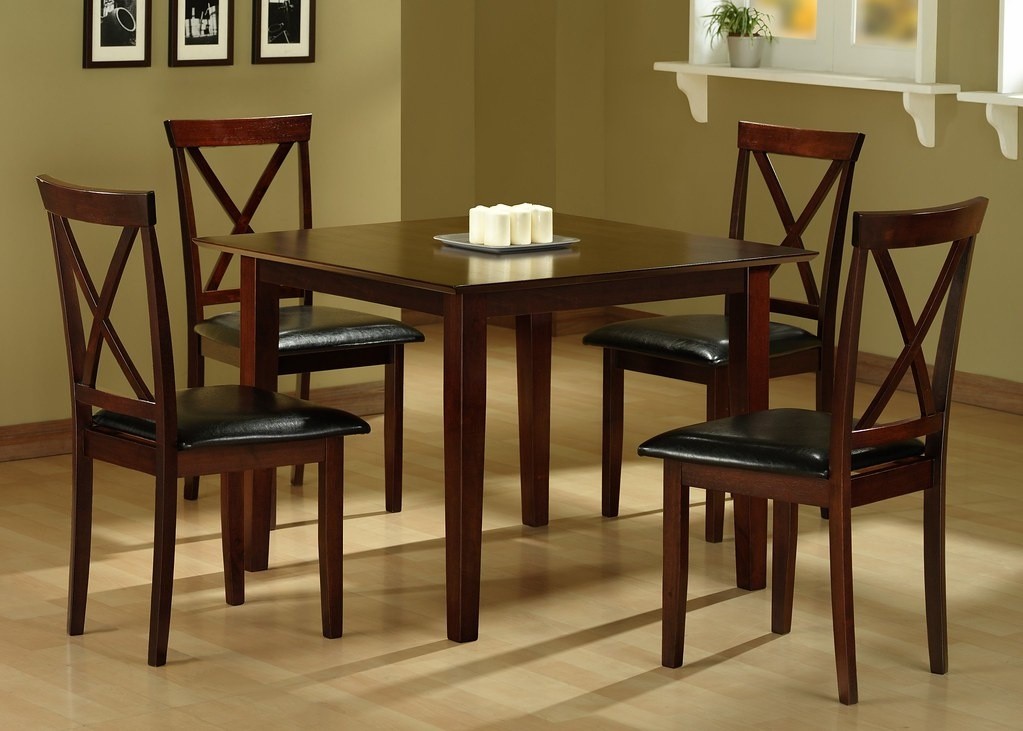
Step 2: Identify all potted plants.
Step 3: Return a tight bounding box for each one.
[700,1,775,68]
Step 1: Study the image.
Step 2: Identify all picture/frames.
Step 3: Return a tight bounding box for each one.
[83,0,152,68]
[168,0,234,65]
[252,0,314,63]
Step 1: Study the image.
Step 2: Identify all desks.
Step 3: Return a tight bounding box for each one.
[192,208,820,645]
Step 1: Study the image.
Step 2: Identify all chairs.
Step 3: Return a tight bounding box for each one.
[30,177,371,664]
[164,110,425,529]
[584,120,866,541]
[635,195,994,703]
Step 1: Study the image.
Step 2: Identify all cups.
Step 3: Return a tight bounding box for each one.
[468,203,554,247]
